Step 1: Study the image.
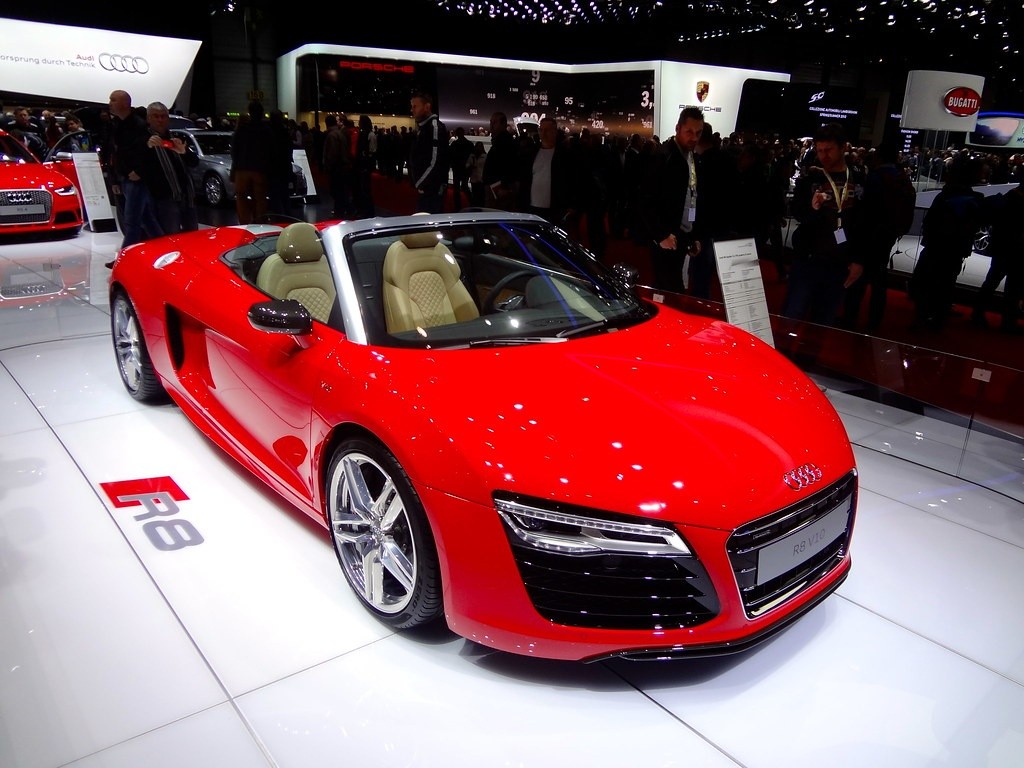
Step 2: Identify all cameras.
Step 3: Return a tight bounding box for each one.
[161,140,173,148]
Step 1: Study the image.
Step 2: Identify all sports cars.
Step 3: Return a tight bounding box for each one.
[106,206,860,665]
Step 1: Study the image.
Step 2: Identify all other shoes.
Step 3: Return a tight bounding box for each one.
[105,260,115,268]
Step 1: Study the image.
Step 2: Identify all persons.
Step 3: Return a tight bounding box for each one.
[10,107,89,161]
[100,91,206,269]
[215,94,1024,336]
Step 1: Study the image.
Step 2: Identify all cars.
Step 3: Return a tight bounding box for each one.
[0,115,238,242]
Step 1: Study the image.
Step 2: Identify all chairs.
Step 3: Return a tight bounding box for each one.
[383,212,480,334]
[257,222,337,325]
